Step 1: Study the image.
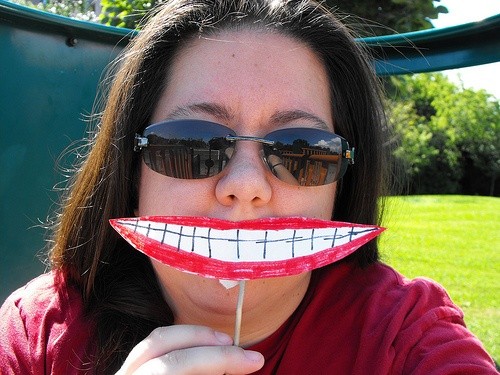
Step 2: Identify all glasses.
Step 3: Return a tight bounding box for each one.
[134,118,355,187]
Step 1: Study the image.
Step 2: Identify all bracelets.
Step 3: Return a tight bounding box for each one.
[271,163,283,168]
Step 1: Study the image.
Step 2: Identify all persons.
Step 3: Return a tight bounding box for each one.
[0,0,500,375]
[266,153,301,187]
[221,138,234,160]
